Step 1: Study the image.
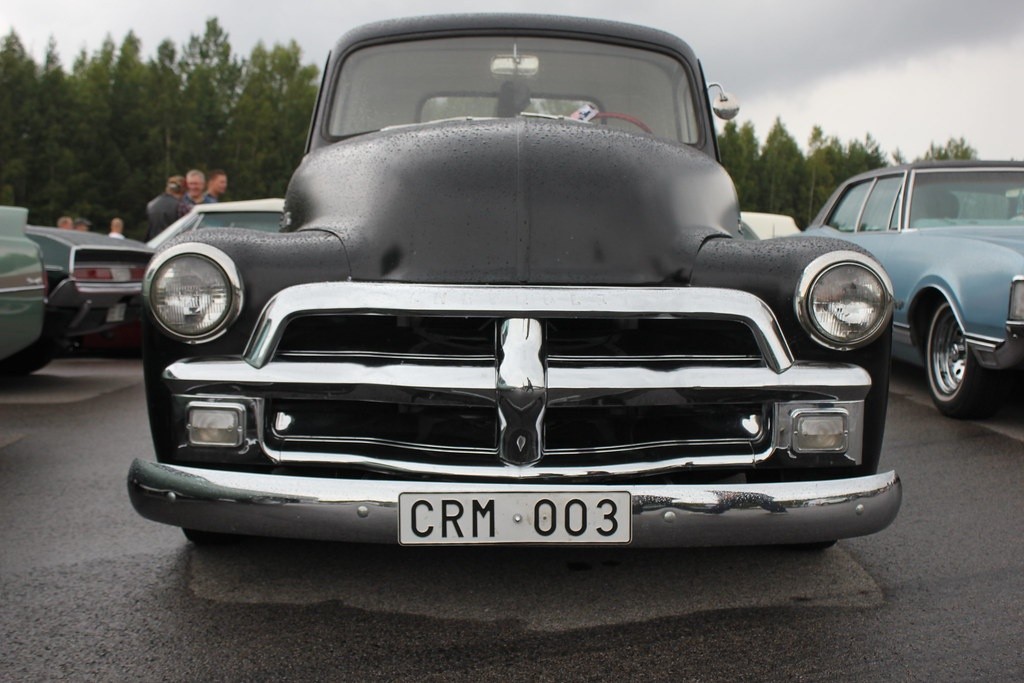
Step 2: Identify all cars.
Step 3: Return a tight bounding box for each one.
[23,230,158,359]
[1,207,50,380]
[142,197,288,258]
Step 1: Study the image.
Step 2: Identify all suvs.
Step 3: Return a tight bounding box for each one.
[798,158,1024,417]
[127,10,903,554]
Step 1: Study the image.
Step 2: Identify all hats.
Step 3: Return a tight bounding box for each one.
[166,175,186,192]
[74,217,91,226]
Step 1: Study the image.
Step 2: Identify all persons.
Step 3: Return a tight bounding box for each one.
[108,218,125,240]
[57,216,74,229]
[145,169,228,242]
[75,217,92,231]
